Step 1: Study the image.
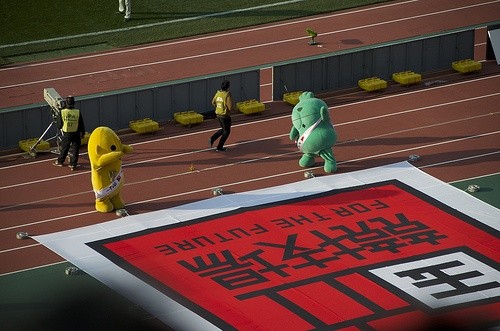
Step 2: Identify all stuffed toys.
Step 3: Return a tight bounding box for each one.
[88,126,133,212]
[289,92,339,173]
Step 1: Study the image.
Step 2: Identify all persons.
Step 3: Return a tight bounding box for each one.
[207,80,233,152]
[53,97,86,171]
[117,0,132,22]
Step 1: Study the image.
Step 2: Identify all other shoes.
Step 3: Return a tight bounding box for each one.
[123,17,130,23]
[115,10,126,15]
[52,160,64,168]
[208,136,215,149]
[67,163,76,172]
[217,145,229,153]
